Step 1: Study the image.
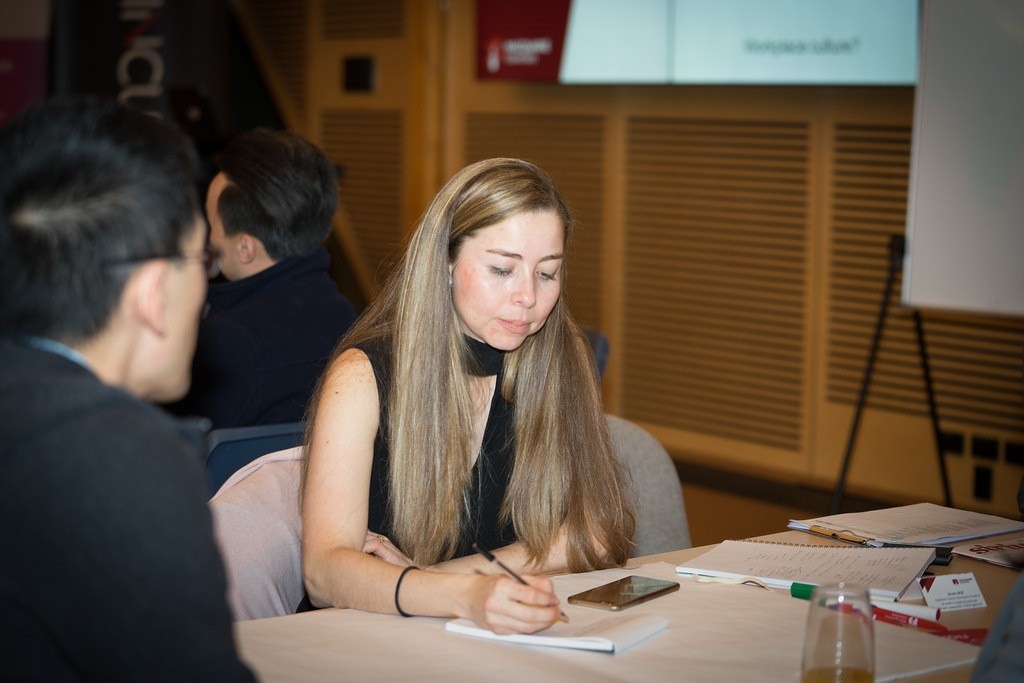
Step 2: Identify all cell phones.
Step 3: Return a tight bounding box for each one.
[568,575,680,611]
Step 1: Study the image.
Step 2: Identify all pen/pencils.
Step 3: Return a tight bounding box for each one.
[471,541,572,625]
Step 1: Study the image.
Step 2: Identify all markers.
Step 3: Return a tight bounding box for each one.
[790,581,950,637]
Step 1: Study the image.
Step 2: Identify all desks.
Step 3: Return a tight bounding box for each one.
[236,530,1020,683]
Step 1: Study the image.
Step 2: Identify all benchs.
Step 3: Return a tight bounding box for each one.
[207,412,691,618]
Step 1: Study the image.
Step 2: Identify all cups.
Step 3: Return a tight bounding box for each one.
[800,583,877,683]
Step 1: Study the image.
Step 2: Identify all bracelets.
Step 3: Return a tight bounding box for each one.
[395,566,420,618]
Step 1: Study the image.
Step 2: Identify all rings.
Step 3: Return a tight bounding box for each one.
[375,533,385,543]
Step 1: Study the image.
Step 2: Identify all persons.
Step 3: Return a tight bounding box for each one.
[967,575,1024,683]
[0,92,259,683]
[294,156,640,636]
[152,129,364,429]
